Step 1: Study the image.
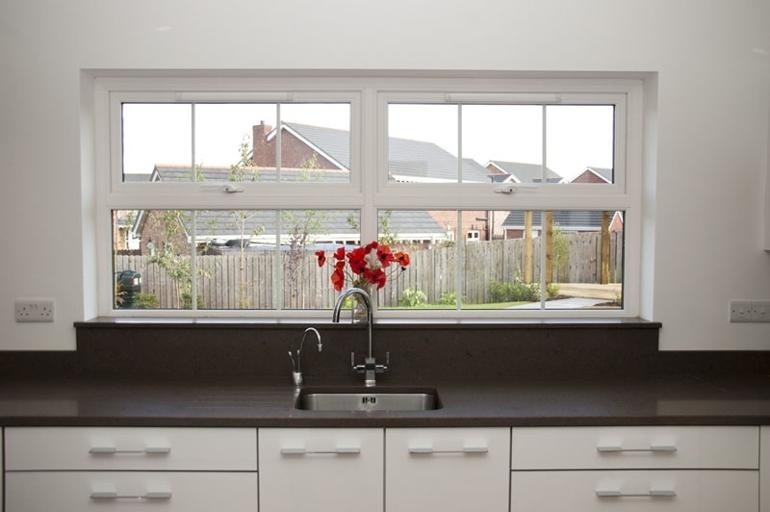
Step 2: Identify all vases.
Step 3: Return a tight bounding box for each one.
[352,281,374,316]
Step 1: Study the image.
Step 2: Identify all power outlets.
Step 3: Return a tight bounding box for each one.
[729,300,770,323]
[14,298,55,323]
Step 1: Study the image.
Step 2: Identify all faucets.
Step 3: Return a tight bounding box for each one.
[287,328,324,388]
[333,287,391,389]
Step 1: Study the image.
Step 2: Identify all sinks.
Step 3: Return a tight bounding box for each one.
[292,387,445,411]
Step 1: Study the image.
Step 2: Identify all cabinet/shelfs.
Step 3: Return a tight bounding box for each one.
[3,424,258,512]
[510,425,759,512]
[385,427,511,512]
[258,427,385,512]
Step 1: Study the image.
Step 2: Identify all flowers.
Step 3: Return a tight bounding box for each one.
[316,240,410,294]
